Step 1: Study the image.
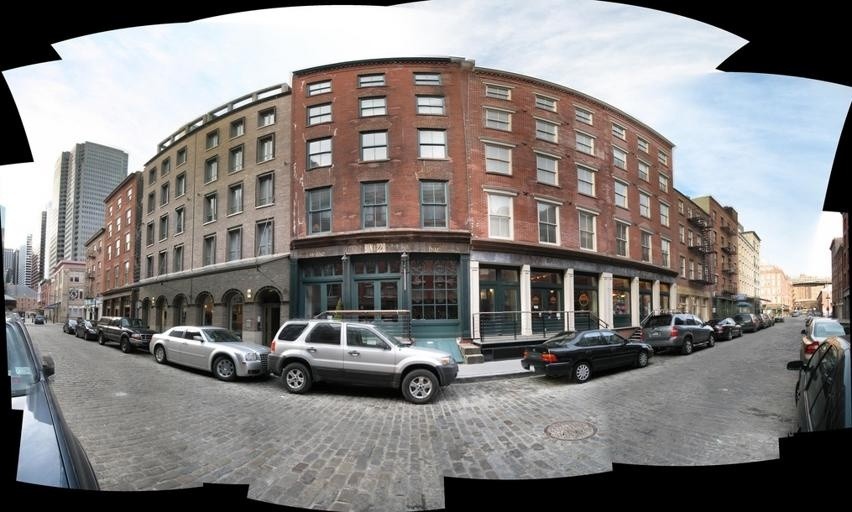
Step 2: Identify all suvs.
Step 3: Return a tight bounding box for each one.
[269,317,459,405]
[97,315,160,353]
[639,309,716,353]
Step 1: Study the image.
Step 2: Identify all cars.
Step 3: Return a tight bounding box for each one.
[6,320,101,491]
[34,315,45,324]
[705,317,743,340]
[520,329,657,385]
[734,310,783,331]
[5,306,27,324]
[148,324,272,381]
[799,318,852,366]
[62,319,82,334]
[74,319,100,341]
[803,304,823,334]
[786,335,852,436]
[790,309,803,317]
